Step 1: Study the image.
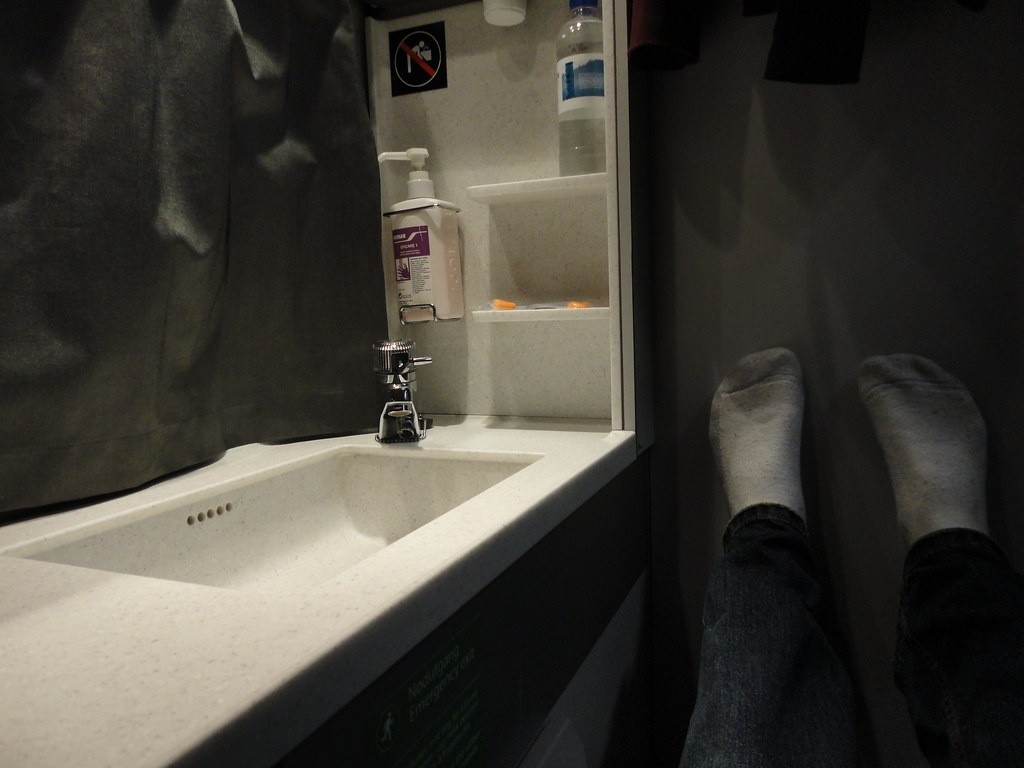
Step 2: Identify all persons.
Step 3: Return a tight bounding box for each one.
[674,344,1024,768]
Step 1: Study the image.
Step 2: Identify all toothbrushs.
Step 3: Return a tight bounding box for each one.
[489,299,589,311]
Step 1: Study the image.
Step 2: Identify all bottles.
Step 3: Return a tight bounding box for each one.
[556,0,608,178]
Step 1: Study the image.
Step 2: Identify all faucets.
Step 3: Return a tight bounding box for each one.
[373,336,433,441]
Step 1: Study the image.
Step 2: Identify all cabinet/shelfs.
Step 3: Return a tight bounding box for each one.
[365,0,634,434]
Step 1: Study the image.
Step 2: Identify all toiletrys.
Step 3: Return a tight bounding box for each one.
[376,148,462,324]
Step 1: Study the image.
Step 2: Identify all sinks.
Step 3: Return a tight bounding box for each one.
[2,445,548,596]
